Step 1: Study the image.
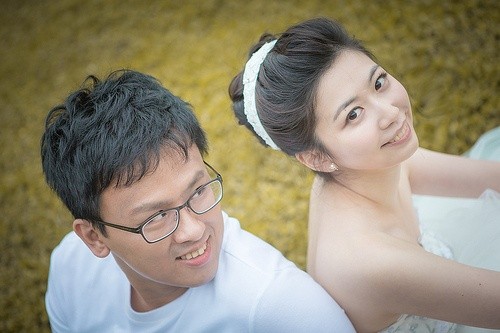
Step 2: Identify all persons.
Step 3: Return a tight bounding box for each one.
[39,68,359,333]
[227,18,500,333]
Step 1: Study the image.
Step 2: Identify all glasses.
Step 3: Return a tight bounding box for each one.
[89,160,224,244]
